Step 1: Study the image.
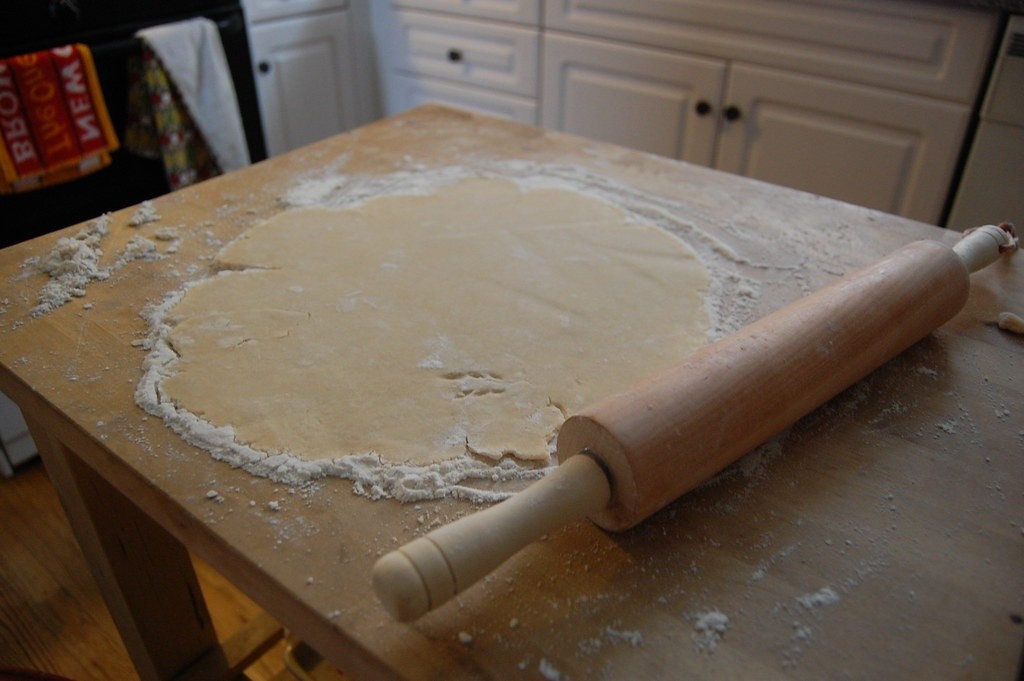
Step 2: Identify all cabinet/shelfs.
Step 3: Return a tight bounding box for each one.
[242,0,376,158]
[369,0,540,135]
[948,14,1024,245]
[540,1,1002,228]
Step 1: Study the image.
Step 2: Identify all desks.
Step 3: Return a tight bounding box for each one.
[0,103,1024,681]
